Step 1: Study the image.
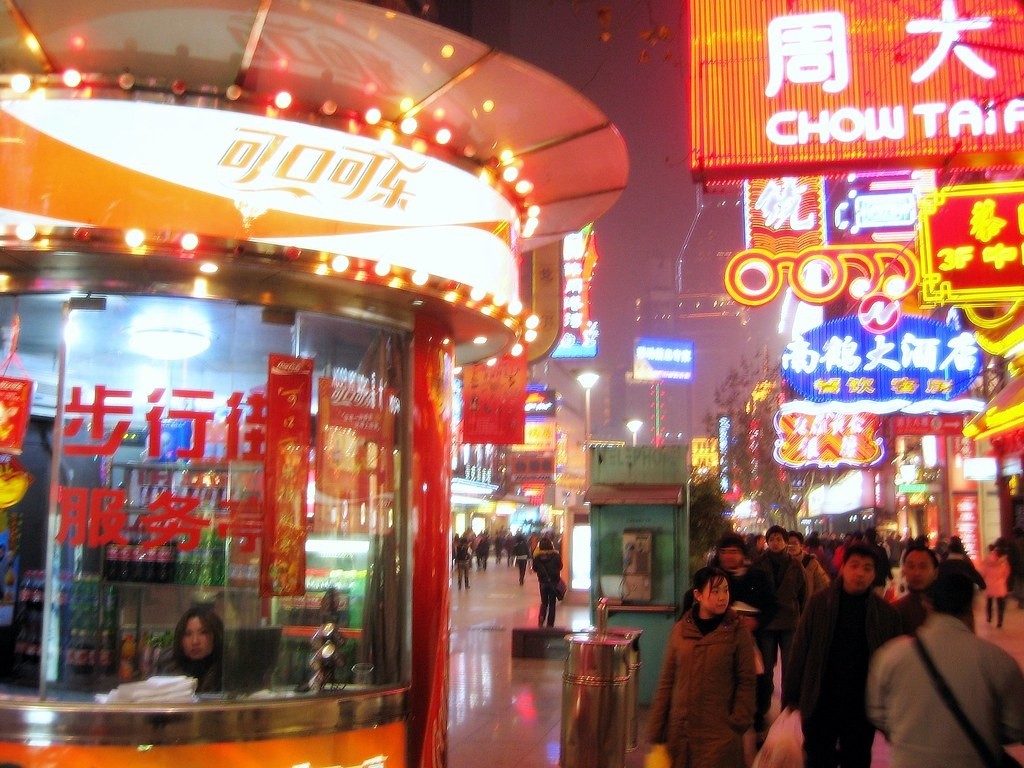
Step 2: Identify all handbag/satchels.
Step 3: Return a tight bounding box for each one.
[749,631,765,675]
[752,707,805,768]
[554,578,568,601]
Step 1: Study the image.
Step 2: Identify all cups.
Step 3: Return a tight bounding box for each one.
[351,663,374,684]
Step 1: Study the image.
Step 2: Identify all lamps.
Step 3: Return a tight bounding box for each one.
[70,293,106,310]
[127,302,211,360]
[261,307,296,326]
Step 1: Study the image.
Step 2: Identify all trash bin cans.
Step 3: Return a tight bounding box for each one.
[559,597,645,768]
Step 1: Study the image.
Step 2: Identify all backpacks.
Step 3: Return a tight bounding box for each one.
[457,545,469,560]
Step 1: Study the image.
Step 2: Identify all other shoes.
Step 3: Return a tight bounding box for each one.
[538,616,545,627]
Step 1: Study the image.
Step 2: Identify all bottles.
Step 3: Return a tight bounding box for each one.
[13,507,370,684]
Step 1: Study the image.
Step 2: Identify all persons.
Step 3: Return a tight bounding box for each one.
[532,537,563,627]
[644,525,1024,768]
[146,605,249,694]
[452,525,563,590]
